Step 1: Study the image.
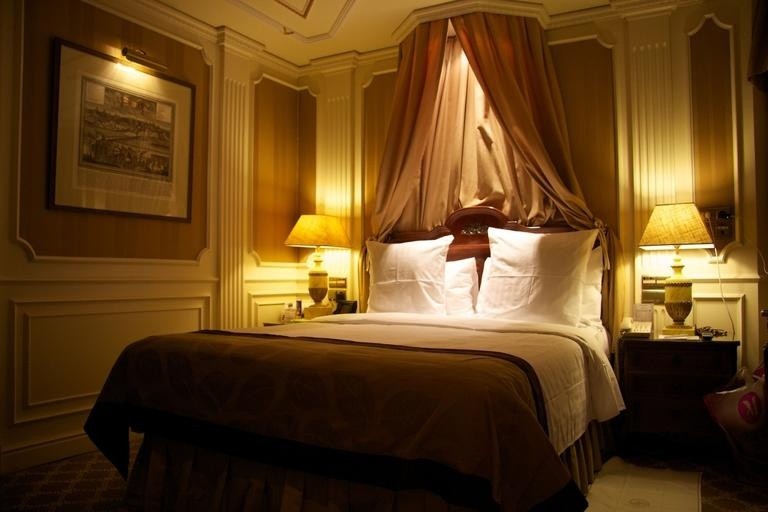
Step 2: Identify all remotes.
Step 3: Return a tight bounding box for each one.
[702,332,713,340]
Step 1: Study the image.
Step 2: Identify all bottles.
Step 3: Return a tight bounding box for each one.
[284,303,297,320]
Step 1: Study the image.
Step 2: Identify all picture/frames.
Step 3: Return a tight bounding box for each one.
[47,36,196,224]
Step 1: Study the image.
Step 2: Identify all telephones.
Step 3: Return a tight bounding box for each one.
[620,317,652,338]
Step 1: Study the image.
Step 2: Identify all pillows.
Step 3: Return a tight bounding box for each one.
[360,226,602,326]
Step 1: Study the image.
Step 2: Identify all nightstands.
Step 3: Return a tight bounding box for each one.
[617,331,740,473]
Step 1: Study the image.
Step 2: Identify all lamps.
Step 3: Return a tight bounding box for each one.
[120,45,169,77]
[284,214,351,320]
[637,202,715,336]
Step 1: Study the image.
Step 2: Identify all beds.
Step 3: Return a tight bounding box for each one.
[114,205,618,510]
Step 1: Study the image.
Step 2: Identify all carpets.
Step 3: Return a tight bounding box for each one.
[698,470,768,512]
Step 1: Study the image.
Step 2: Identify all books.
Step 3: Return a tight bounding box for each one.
[658,335,700,341]
